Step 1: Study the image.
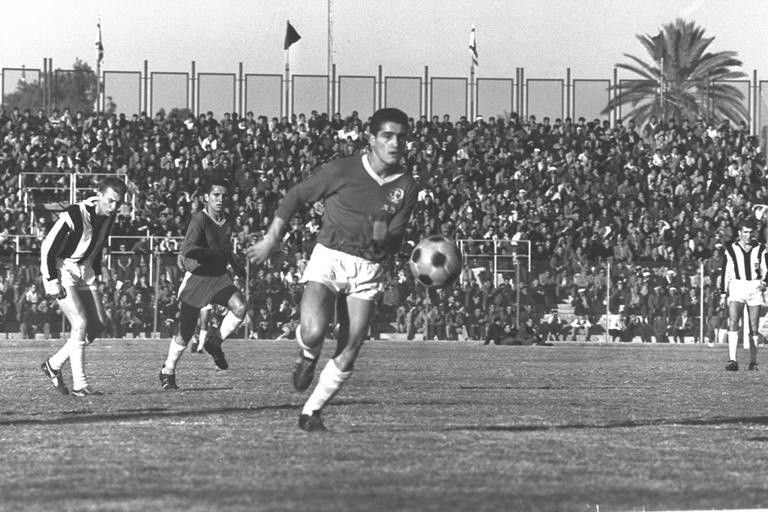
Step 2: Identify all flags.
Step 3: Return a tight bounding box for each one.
[465,26,479,75]
[282,24,302,51]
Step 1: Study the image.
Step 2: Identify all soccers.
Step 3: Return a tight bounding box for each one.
[411,235,462,288]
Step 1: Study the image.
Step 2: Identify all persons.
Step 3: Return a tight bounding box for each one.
[716,220,768,371]
[0,101,768,346]
[157,178,252,391]
[240,105,421,435]
[38,175,130,397]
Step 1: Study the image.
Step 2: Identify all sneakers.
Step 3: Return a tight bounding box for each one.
[40,357,69,397]
[190,338,200,354]
[748,362,760,370]
[724,359,738,371]
[298,409,326,430]
[203,331,229,369]
[293,350,320,392]
[159,364,180,391]
[71,387,107,396]
